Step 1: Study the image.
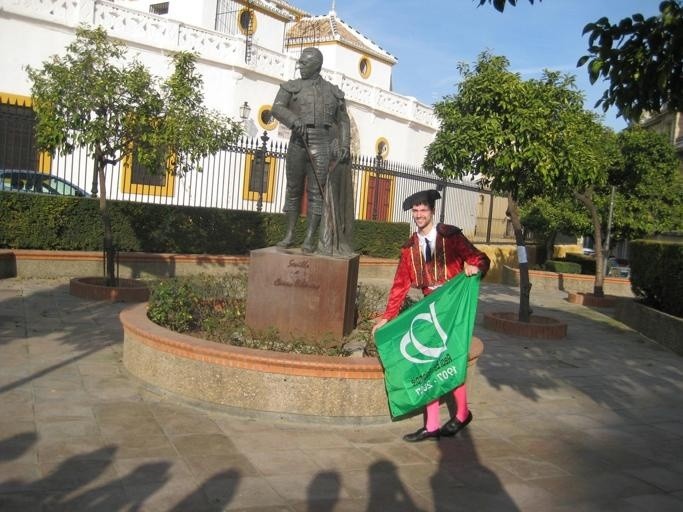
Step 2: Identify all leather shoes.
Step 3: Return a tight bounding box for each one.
[404,427,440,442]
[440,410,472,436]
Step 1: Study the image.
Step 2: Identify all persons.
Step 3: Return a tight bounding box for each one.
[371,189,490,442]
[271,47,350,254]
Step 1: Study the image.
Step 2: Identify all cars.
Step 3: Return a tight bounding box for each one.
[609,257,632,279]
[582,247,596,259]
[1,169,94,198]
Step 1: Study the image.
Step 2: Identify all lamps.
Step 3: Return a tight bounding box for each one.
[231,102,251,123]
